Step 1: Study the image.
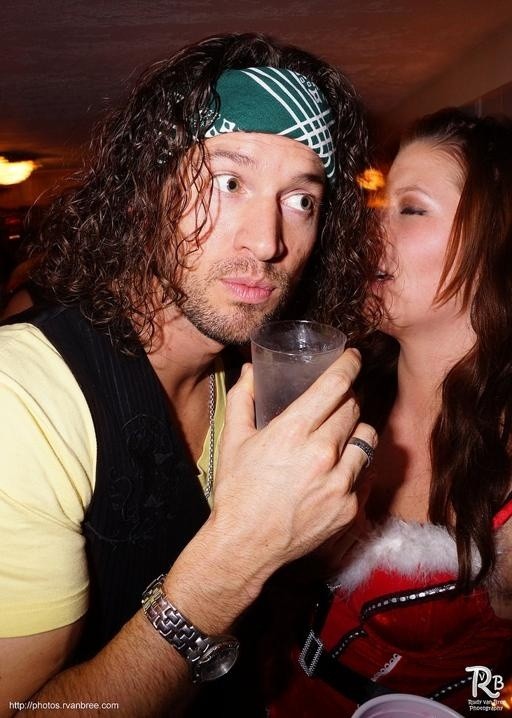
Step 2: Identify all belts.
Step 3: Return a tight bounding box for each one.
[296,630,399,708]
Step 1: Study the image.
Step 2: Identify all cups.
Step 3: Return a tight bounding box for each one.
[249,319,348,431]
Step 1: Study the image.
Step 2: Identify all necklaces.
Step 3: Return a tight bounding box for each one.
[202,368,218,500]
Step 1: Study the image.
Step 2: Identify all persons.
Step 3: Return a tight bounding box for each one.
[257,103,512,717]
[1,272,47,321]
[6,248,47,290]
[1,33,380,716]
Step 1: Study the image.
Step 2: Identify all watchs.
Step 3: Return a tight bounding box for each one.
[140,571,241,686]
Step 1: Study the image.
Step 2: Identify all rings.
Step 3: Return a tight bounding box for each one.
[345,434,376,469]
